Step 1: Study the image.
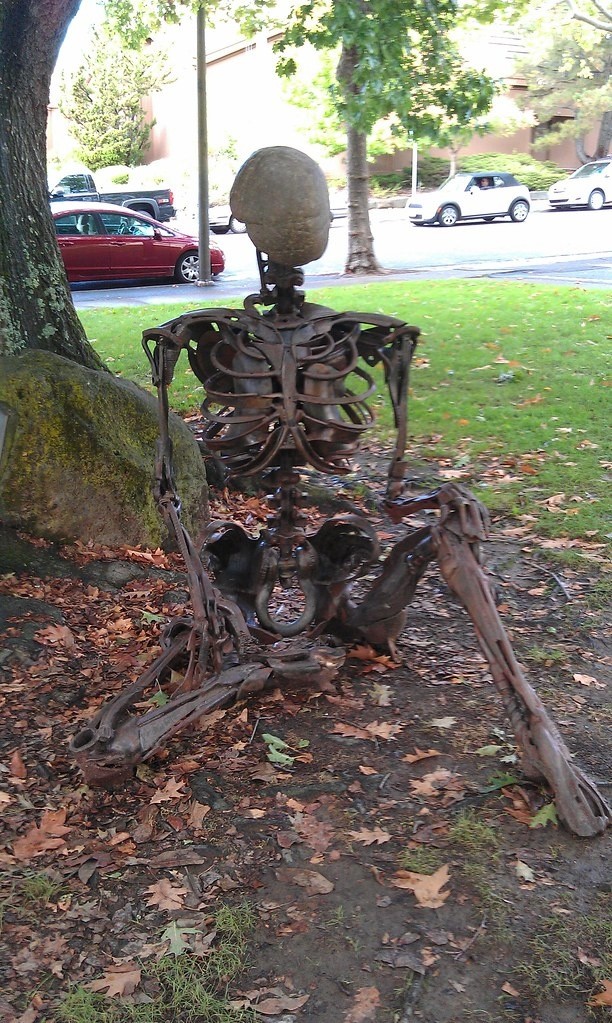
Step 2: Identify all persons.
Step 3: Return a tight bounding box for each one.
[479,177,490,187]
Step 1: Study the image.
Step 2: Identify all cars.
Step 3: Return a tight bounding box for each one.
[406,171,532,228]
[203,190,348,234]
[548,155,612,211]
[46,200,227,283]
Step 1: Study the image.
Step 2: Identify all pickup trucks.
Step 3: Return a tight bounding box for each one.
[43,171,176,226]
